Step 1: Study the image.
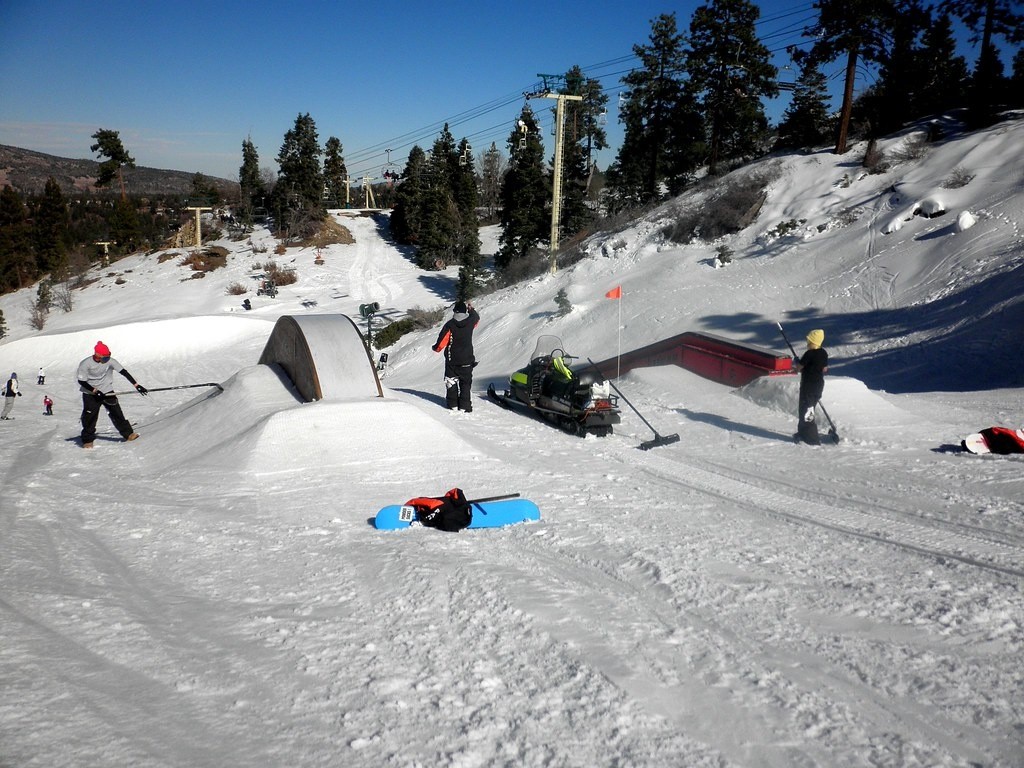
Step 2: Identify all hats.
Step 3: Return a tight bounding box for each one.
[11,373,17,379]
[453,301,466,314]
[805,329,824,347]
[94,341,111,356]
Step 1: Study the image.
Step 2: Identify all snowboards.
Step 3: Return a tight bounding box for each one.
[375,498,542,533]
[965,428,1024,455]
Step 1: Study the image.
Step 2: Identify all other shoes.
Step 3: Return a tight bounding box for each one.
[83,442,92,449]
[792,432,820,445]
[127,433,139,441]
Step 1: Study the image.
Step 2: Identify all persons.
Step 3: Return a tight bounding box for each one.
[432,301,480,413]
[43,396,54,415]
[37,367,45,385]
[1,372,22,420]
[385,169,398,181]
[77,341,148,448]
[793,329,829,446]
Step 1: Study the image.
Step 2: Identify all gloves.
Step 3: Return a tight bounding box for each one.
[136,384,148,396]
[95,390,105,399]
[1,392,5,396]
[17,392,22,396]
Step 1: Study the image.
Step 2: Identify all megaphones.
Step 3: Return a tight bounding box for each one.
[359,302,380,318]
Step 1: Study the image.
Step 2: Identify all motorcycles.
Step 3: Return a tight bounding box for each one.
[488,335,622,439]
